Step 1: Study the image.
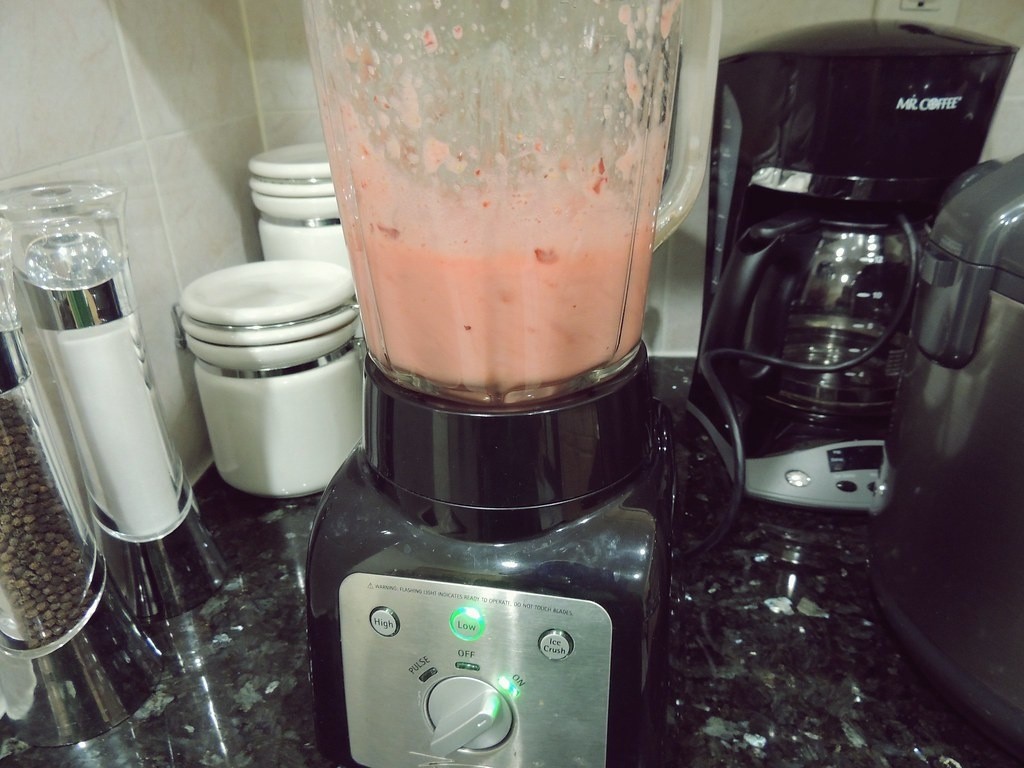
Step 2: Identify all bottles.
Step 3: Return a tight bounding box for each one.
[177,257,366,500]
[0,191,164,748]
[5,176,228,618]
[249,143,346,261]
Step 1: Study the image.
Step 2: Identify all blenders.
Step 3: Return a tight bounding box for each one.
[305,0,680,766]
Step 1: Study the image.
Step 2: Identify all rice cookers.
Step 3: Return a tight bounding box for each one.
[871,154,1022,756]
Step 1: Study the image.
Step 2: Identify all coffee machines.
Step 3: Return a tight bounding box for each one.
[687,15,1024,512]
